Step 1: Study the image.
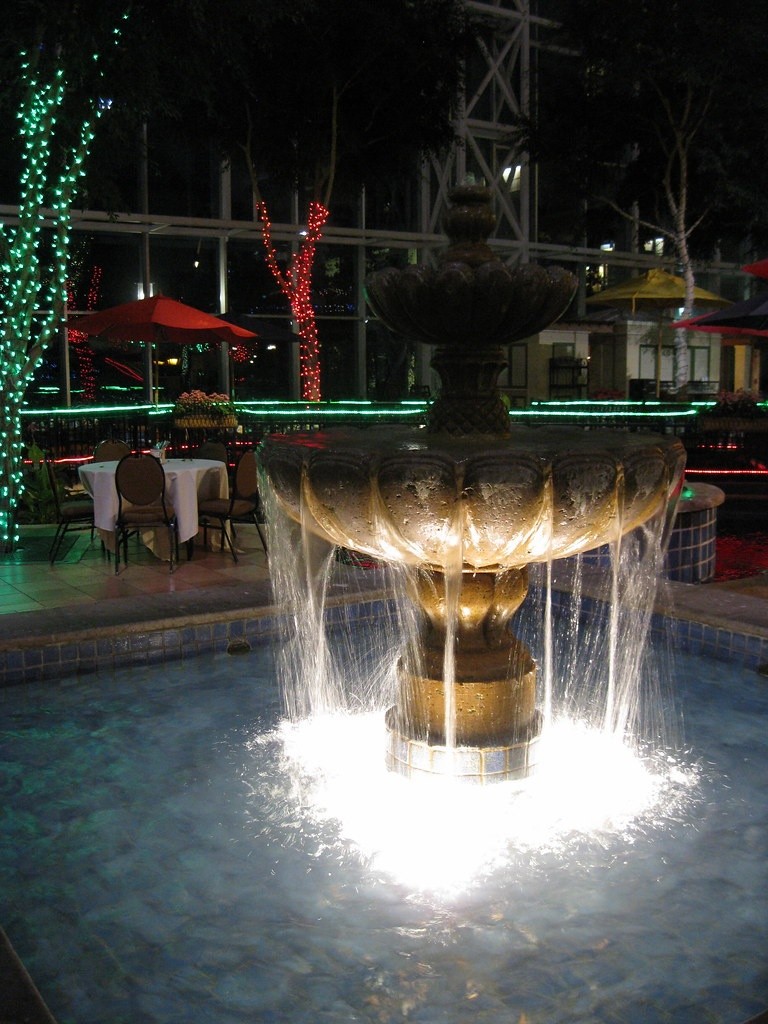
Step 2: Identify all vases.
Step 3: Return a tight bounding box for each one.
[174,409,238,428]
[697,415,768,431]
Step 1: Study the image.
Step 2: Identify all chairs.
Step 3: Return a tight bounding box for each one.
[189,449,266,563]
[46,462,111,564]
[113,451,181,575]
[198,439,229,464]
[94,439,131,462]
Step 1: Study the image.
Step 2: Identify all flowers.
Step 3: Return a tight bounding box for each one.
[174,389,239,414]
[712,387,763,416]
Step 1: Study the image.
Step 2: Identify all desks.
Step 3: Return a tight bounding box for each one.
[78,458,231,561]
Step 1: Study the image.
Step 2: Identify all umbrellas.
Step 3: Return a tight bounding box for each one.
[579,267,768,405]
[75,288,256,405]
